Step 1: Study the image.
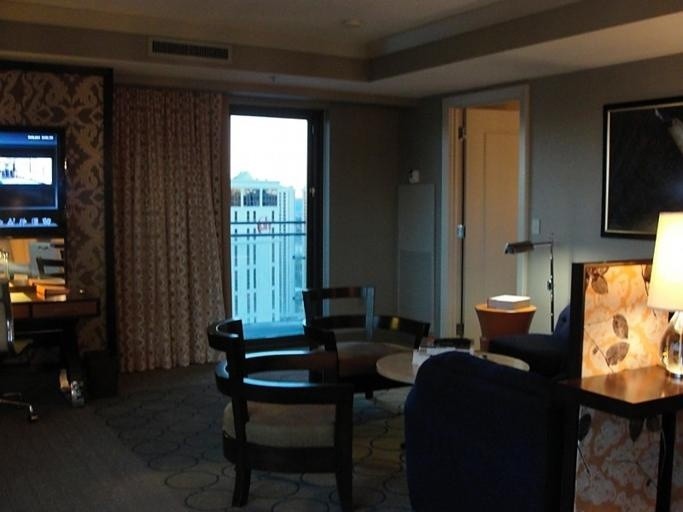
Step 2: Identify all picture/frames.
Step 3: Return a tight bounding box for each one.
[600,95,682,240]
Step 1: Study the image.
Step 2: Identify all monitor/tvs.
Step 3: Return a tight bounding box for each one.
[0,123,67,226]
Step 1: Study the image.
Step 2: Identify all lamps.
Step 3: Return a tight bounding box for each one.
[504,240,555,335]
[647,212,682,378]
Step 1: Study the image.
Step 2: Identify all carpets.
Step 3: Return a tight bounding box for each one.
[94,382,413,511]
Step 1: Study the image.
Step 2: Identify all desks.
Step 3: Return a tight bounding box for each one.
[11,289,101,408]
[560,365,682,512]
[377,347,531,386]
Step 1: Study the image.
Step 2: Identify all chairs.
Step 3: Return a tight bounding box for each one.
[397,306,571,511]
[205,318,355,512]
[1,282,41,423]
[301,285,431,385]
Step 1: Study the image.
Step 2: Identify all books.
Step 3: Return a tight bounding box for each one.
[410,348,481,369]
[418,336,474,357]
[487,294,530,310]
[21,240,68,298]
